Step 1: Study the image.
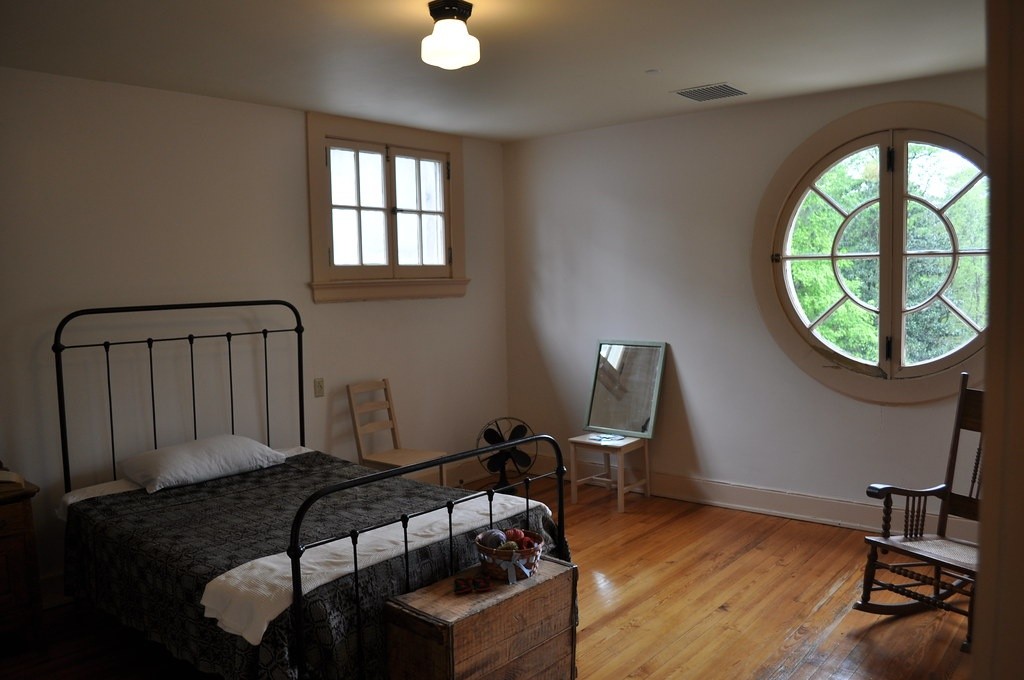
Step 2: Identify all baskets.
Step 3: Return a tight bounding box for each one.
[474,528,544,580]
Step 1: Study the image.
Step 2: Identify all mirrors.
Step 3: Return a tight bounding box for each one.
[581,339,665,439]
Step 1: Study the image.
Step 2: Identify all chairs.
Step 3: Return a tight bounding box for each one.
[853,371,985,654]
[568,432,651,514]
[347,378,447,486]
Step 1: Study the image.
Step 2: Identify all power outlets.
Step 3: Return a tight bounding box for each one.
[314,378,324,397]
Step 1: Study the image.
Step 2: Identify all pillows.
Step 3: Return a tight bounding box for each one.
[116,434,285,494]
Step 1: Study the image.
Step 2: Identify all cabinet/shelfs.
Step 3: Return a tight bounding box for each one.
[383,553,580,680]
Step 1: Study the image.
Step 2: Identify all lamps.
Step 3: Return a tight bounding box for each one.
[420,0,482,71]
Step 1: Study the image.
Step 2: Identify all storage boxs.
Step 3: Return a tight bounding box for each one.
[474,529,545,581]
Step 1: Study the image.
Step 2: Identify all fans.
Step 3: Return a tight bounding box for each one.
[476,417,538,496]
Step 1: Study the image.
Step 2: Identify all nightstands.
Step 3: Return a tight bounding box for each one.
[0,479,51,655]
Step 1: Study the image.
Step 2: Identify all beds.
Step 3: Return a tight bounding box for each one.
[50,299,567,680]
